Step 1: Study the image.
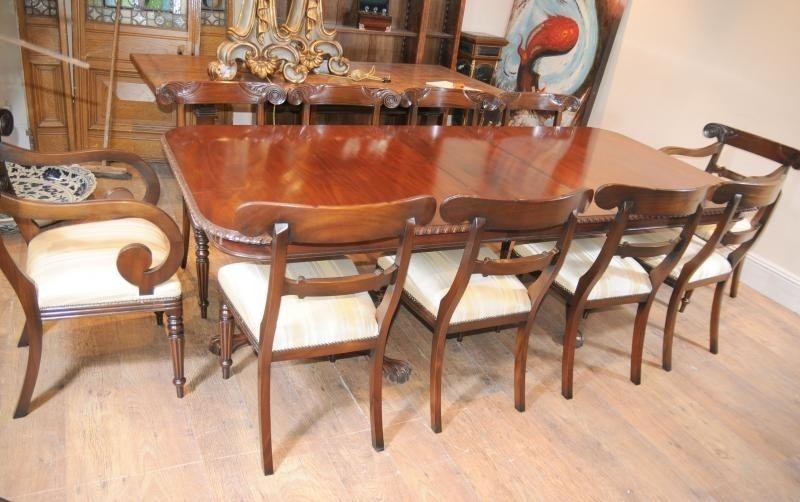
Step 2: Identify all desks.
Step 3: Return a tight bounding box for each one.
[131,53,507,127]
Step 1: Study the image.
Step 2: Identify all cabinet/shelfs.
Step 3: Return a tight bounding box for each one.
[253,0,465,124]
